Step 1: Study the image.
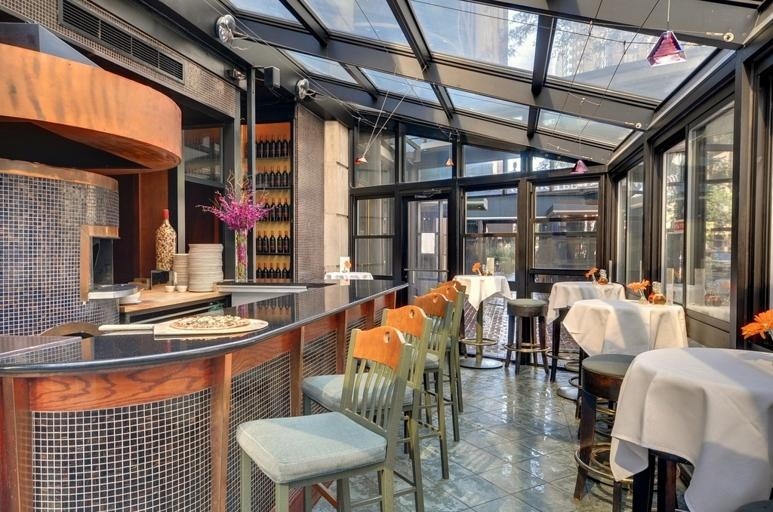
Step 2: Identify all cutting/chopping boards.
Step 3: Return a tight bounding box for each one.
[98,317,271,338]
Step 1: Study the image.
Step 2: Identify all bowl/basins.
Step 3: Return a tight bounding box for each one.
[164,284,187,295]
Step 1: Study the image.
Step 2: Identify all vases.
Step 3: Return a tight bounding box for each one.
[234,229,247,285]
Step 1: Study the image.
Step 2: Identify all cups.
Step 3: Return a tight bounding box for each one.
[340,256,350,273]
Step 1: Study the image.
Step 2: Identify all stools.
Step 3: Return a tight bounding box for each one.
[503,299,551,378]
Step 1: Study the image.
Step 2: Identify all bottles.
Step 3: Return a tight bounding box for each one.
[255,196,288,222]
[242,163,288,188]
[256,229,289,253]
[703,278,722,307]
[480,263,489,276]
[598,269,608,284]
[647,282,667,305]
[663,284,674,306]
[244,135,289,157]
[256,261,291,279]
[154,207,176,272]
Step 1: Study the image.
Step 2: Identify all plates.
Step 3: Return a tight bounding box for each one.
[186,172,209,179]
[171,244,225,293]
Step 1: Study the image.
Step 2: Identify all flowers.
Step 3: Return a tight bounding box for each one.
[194,168,280,279]
[741,308,773,343]
[625,279,651,303]
[585,267,599,281]
[471,262,484,275]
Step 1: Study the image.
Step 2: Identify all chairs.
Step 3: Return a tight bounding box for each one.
[404,281,471,412]
[573,353,646,511]
[385,286,466,442]
[301,304,434,512]
[236,326,414,512]
[359,293,454,479]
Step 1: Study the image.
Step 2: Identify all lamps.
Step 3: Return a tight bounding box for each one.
[645,2,686,68]
[570,120,590,177]
[443,133,454,166]
[356,60,427,167]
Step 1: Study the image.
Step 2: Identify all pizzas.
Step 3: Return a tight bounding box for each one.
[169,314,251,331]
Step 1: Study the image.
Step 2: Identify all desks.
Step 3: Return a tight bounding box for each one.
[452,276,512,368]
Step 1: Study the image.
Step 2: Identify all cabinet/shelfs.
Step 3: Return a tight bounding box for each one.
[241,123,295,279]
